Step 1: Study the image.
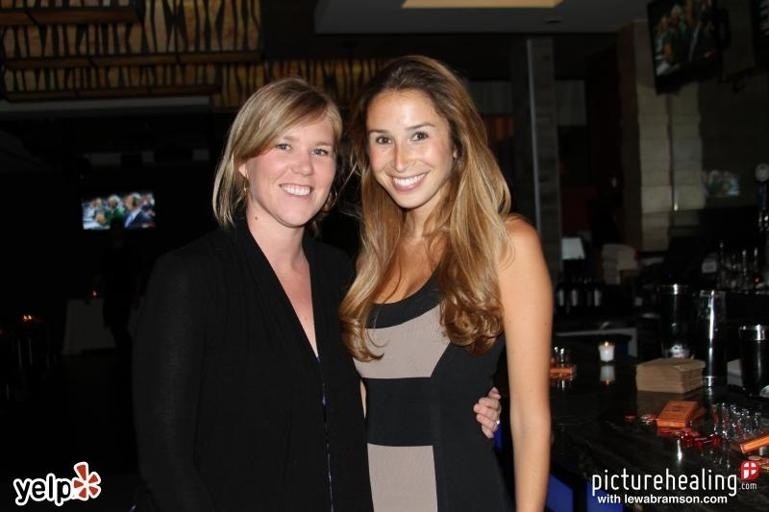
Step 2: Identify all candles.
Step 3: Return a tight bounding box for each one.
[598,342,615,362]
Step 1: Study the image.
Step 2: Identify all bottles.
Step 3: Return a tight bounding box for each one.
[718,248,764,294]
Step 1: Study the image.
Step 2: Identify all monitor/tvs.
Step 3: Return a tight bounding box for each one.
[80,186,160,238]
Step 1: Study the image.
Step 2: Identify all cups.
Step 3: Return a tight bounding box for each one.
[738,324,767,393]
[655,284,727,383]
[598,340,615,362]
[553,346,571,365]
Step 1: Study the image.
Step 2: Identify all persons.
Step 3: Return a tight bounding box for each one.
[132,75,502,512]
[338,55,553,512]
[89,193,154,229]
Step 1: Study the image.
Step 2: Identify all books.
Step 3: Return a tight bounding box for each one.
[602,244,639,285]
[635,358,706,394]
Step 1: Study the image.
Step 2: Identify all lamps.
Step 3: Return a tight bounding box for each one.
[561,238,585,261]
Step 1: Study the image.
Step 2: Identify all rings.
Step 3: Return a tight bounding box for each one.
[496,416,500,425]
